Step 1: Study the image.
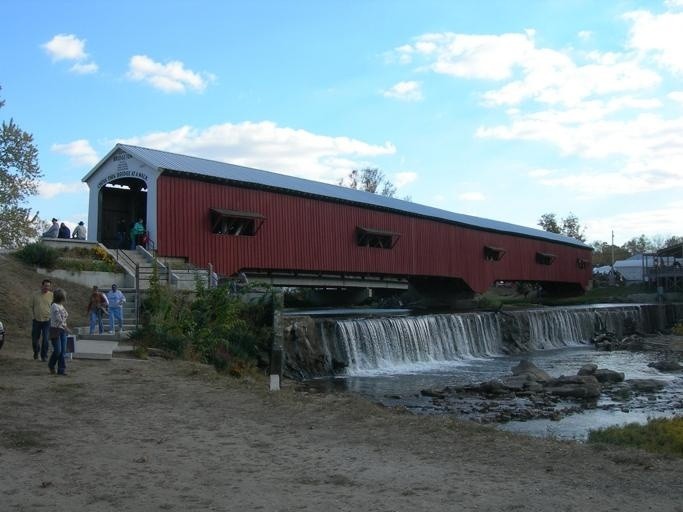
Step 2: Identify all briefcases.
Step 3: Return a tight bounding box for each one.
[65,333,77,353]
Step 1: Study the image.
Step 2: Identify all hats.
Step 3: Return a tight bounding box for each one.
[77,221,84,224]
[51,218,57,222]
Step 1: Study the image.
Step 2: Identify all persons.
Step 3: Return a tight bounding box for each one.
[104,283,126,333]
[71,220,87,240]
[27,277,53,362]
[114,216,126,249]
[47,286,69,374]
[86,283,109,336]
[134,217,145,245]
[125,216,136,248]
[41,218,59,238]
[57,223,71,239]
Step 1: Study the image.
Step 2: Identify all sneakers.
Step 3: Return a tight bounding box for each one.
[31,352,50,362]
[107,329,124,334]
[48,366,70,376]
